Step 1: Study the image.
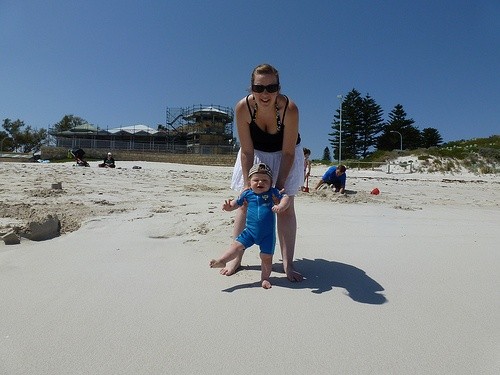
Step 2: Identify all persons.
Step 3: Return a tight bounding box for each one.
[315,164,347,194]
[98,152,116,168]
[299,147,311,192]
[218,64,304,282]
[209,163,291,289]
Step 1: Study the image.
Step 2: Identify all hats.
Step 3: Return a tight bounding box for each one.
[247,163,273,178]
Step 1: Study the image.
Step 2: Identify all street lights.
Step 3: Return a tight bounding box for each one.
[337,95,343,164]
[391,130,403,150]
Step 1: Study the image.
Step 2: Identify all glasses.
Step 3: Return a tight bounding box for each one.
[251,80,280,93]
